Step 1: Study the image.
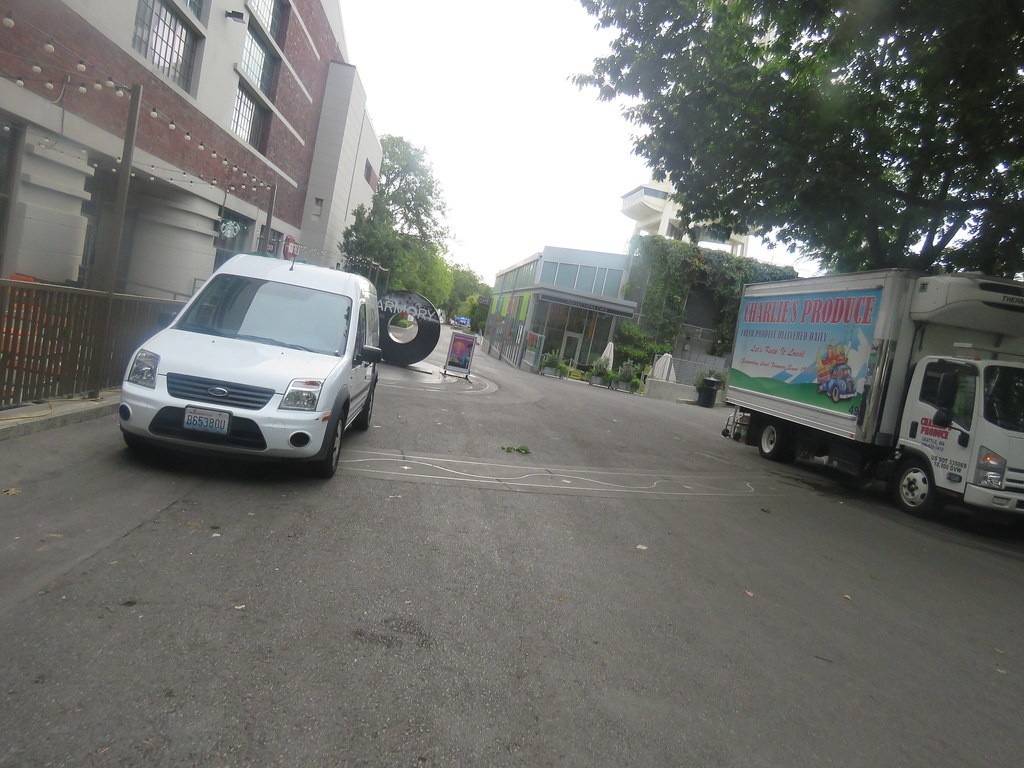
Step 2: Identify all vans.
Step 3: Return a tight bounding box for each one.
[117,252,383,481]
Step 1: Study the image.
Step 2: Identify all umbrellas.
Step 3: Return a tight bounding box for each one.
[645,353,677,383]
[596,341,614,371]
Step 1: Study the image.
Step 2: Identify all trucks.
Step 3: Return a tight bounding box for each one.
[722,266,1024,531]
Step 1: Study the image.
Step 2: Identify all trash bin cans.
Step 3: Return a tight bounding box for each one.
[697,376,721,408]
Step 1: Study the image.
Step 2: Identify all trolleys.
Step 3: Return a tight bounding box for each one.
[721,405,745,441]
